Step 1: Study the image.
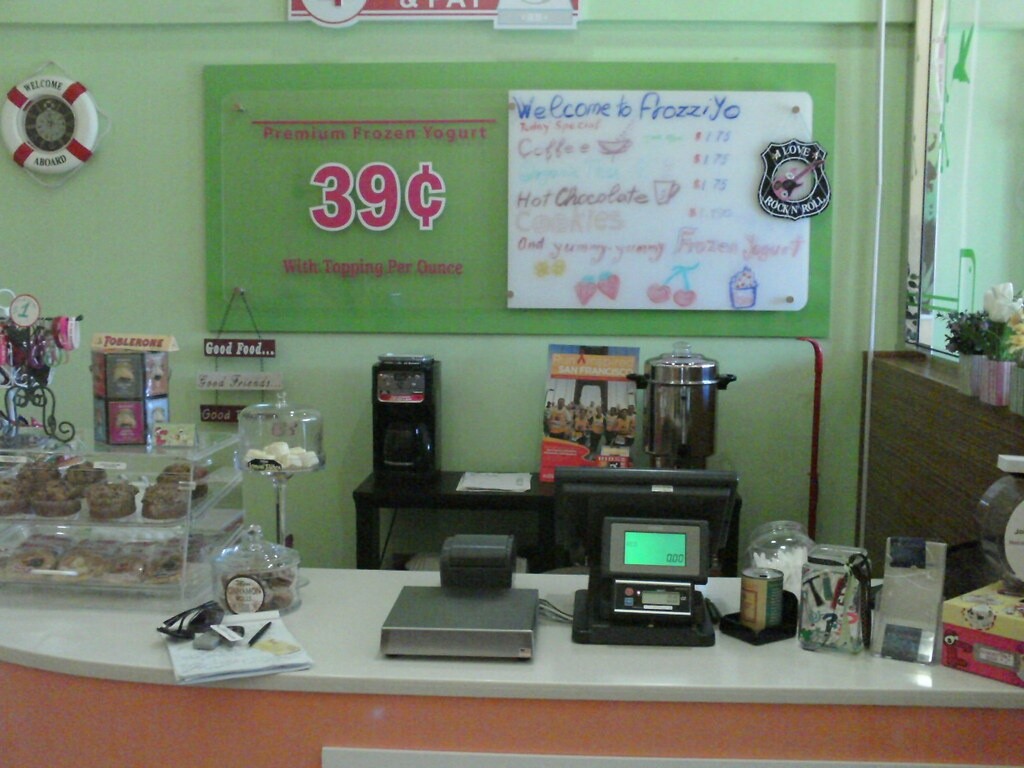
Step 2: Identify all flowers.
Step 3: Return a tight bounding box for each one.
[935,308,990,355]
[1005,308,1024,368]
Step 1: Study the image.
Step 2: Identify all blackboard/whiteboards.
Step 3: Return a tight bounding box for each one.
[504,86,816,314]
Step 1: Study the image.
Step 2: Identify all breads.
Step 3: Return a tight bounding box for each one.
[0,533,213,587]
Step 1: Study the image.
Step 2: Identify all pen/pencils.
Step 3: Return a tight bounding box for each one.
[247,620,273,647]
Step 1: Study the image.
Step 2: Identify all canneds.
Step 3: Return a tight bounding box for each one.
[739,568,784,631]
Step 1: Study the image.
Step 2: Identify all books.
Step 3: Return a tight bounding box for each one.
[159,609,313,687]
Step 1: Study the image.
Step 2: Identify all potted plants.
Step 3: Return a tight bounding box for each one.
[978,319,1016,406]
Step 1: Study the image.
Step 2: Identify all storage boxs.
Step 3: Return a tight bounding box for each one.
[940,580,1024,687]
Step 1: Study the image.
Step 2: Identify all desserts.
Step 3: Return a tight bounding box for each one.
[244,440,319,470]
[0,453,211,521]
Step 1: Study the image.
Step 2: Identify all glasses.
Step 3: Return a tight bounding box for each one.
[156,601,225,640]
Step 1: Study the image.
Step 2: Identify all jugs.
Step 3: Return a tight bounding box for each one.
[381,417,434,467]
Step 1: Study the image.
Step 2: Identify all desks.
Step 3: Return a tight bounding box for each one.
[353,472,743,577]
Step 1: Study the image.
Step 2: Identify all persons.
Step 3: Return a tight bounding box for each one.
[543,397,637,452]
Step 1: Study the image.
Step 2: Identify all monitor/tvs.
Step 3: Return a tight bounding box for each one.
[553,465,739,556]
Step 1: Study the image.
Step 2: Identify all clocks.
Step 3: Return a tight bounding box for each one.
[0,62,112,190]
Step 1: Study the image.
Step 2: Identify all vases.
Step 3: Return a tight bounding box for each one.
[1008,365,1024,416]
[956,352,988,397]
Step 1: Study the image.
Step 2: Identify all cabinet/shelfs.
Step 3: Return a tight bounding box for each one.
[0,437,246,609]
